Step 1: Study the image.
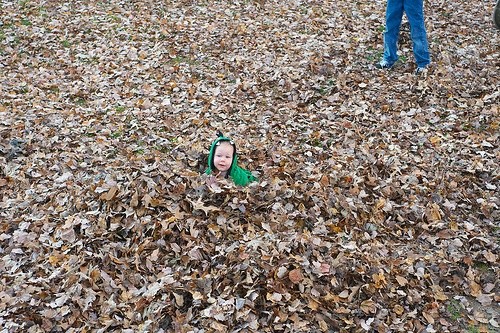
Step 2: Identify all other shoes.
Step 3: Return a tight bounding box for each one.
[414,67,429,82]
[373,59,392,71]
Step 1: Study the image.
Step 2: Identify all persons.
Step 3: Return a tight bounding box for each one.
[202,135,261,188]
[375,0,432,77]
